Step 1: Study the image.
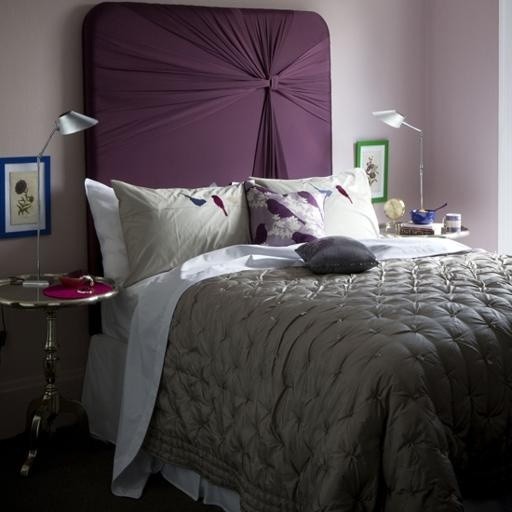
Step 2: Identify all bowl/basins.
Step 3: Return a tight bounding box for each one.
[410,208,436,224]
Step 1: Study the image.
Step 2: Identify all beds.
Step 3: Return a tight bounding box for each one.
[85,227,511,507]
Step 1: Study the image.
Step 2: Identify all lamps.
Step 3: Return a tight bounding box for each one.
[21,108,98,288]
[372,103,433,235]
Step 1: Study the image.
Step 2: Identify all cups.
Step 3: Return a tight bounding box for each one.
[443,213,461,233]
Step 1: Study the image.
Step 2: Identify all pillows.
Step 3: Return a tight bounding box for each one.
[82,168,395,272]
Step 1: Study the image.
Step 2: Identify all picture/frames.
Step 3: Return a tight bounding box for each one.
[1,157,51,238]
[355,140,388,202]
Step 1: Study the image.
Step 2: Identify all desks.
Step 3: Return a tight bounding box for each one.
[2,277,117,478]
